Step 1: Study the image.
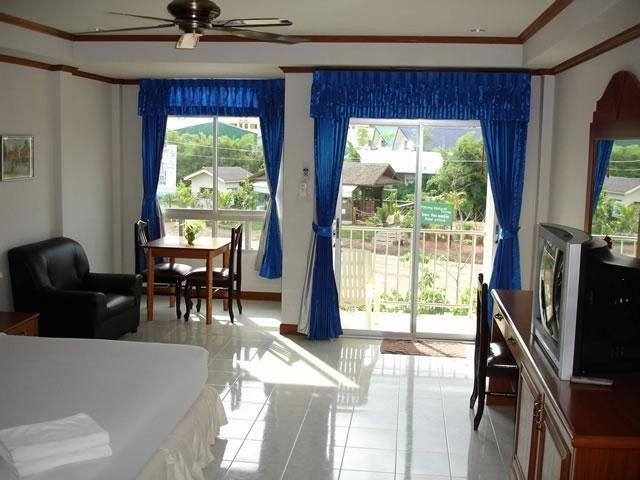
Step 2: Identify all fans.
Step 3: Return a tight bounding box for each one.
[73,0,312,51]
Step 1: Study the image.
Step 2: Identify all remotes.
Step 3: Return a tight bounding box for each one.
[570,376,614,387]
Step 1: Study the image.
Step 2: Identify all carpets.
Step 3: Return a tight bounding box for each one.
[380,338,467,358]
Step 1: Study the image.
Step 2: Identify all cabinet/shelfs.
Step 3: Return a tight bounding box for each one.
[487,288,640,480]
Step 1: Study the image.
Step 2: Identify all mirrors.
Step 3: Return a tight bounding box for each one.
[584,69,640,258]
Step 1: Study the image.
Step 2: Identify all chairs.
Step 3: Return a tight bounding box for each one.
[469,273,519,431]
[7,236,144,340]
[134,219,247,324]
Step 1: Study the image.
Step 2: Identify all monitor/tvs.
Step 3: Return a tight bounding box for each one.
[531,221,640,381]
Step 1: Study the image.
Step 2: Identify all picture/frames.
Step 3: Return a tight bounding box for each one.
[0,135,35,183]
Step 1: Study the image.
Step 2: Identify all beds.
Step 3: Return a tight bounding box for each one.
[0,332,221,480]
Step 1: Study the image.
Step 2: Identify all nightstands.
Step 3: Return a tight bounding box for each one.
[0,310,41,337]
